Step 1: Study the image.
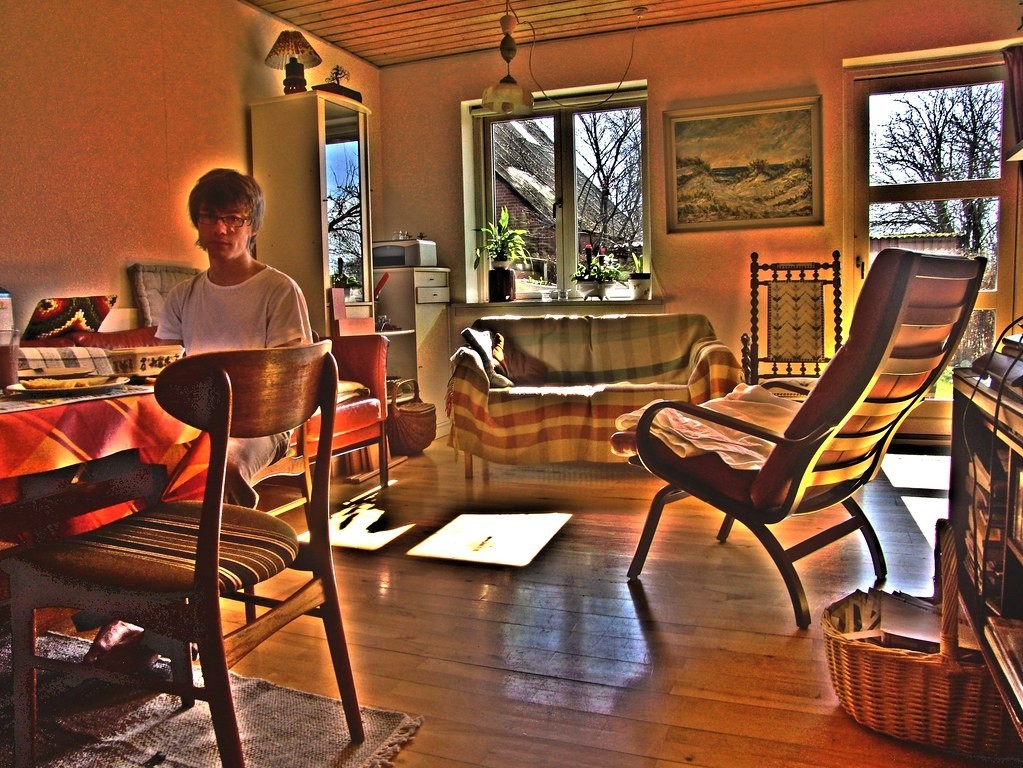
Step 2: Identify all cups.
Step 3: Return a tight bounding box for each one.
[0,330,20,394]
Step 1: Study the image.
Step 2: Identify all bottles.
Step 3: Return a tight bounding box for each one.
[392,229,409,240]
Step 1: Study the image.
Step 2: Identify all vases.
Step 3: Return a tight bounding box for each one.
[629,273,651,279]
[628,279,651,300]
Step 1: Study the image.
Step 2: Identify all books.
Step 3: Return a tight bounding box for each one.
[1001,333,1023,361]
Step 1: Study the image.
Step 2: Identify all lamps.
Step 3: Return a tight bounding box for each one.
[264,29,322,94]
[480,0,536,116]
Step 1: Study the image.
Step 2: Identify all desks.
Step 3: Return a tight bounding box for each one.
[0,384,201,545]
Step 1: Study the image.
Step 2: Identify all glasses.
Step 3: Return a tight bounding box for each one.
[195,212,252,227]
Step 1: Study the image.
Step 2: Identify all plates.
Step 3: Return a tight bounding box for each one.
[17,368,94,381]
[6,377,130,397]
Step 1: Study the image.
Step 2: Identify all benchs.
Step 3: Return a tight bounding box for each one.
[22,330,389,490]
[445,314,747,480]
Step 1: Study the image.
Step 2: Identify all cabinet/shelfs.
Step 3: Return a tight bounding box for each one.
[947,368,1023,740]
[374,268,452,440]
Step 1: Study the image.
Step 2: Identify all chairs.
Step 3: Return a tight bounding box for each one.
[740,249,843,402]
[0,337,352,768]
[249,330,324,530]
[608,248,992,629]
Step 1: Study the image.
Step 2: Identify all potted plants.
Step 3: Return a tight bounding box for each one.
[470,205,532,270]
[312,65,362,104]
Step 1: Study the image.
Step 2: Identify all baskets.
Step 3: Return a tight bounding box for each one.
[821,518,1023,759]
[386,377,436,454]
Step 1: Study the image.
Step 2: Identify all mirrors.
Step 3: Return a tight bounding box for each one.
[316,89,373,333]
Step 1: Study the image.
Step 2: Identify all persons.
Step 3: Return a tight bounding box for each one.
[80,167,315,680]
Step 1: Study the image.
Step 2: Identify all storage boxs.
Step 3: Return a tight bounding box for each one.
[329,288,346,336]
[335,318,373,335]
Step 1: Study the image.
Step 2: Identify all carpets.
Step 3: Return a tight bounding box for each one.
[0,627,427,768]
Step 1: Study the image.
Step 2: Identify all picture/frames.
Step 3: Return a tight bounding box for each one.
[662,91,827,237]
